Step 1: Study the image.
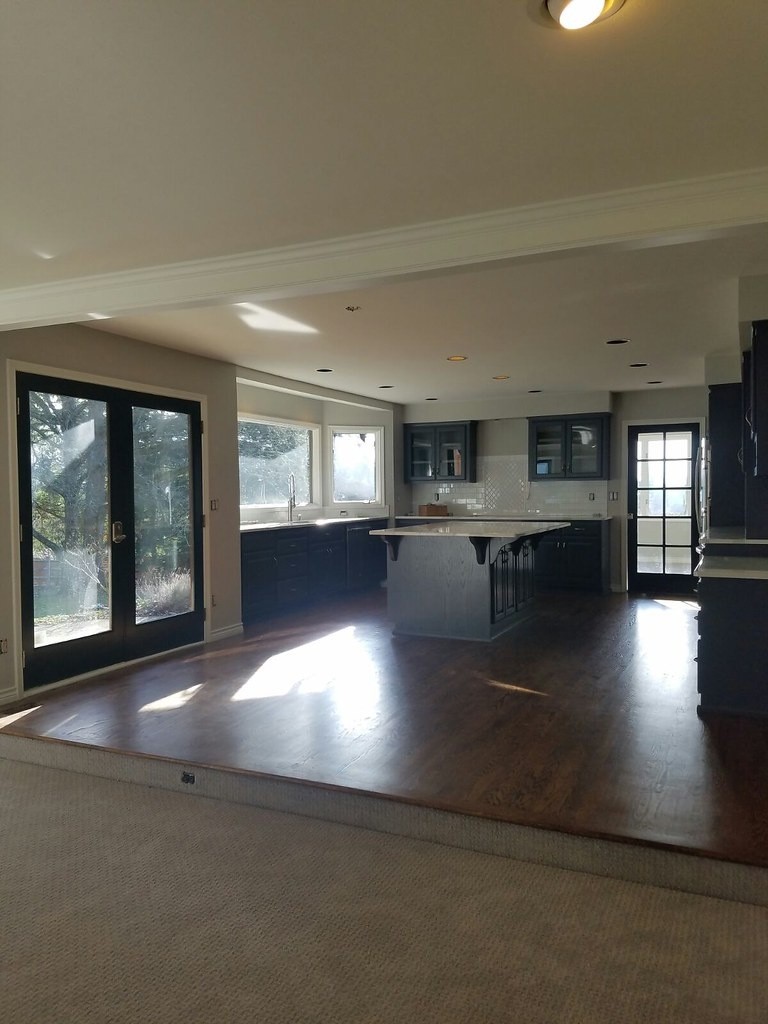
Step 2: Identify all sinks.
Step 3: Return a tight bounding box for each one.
[292,520,320,523]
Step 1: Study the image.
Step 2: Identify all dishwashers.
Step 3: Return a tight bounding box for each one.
[345,524,377,590]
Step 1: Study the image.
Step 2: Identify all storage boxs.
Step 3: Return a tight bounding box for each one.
[419,505,448,516]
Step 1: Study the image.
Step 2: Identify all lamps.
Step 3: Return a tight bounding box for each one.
[546,0,605,32]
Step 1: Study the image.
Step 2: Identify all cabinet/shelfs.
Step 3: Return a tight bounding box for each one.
[367,521,572,642]
[533,520,611,593]
[736,319,768,540]
[403,422,477,484]
[693,578,768,721]
[526,417,611,481]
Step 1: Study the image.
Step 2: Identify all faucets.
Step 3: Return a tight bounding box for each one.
[288,473,296,522]
